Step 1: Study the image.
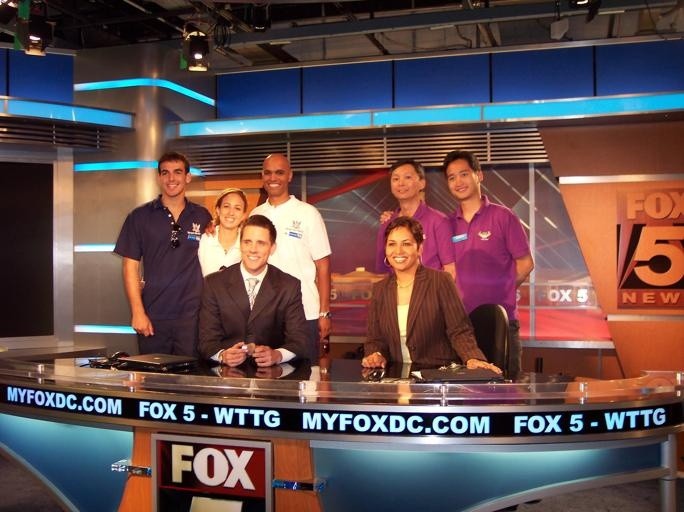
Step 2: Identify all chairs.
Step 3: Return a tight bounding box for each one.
[469,303,510,380]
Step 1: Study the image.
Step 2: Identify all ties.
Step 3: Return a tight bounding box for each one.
[246,278,259,311]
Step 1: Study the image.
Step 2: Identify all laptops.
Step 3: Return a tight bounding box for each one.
[413,368,505,383]
[117,353,199,372]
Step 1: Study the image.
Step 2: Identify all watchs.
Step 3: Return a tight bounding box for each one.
[319,311,333,318]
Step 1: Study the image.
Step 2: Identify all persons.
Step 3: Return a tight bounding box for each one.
[112,152,213,357]
[361,213,503,377]
[380,151,534,377]
[197,188,250,279]
[198,216,318,369]
[209,362,296,379]
[376,158,457,280]
[205,152,333,368]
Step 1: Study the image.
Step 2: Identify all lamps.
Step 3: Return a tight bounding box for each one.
[183,32,209,71]
[25,18,49,57]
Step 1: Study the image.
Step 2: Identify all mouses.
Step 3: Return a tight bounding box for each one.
[369,367,386,379]
[112,351,130,361]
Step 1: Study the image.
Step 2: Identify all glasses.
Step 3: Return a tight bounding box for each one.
[170,224,182,249]
